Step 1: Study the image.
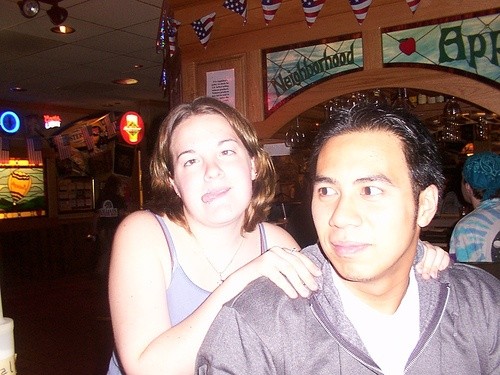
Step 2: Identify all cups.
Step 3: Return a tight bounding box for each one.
[0,318,17,375]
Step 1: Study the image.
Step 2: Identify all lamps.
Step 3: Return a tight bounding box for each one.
[17,0,40,19]
[45,2,68,25]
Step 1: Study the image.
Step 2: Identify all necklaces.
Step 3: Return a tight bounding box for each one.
[186,225,247,287]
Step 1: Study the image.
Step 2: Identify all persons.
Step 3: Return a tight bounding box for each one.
[89,148,500,283]
[195,104,500,375]
[107,93,450,375]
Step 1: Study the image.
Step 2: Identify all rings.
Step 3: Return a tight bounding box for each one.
[291,247,296,254]
